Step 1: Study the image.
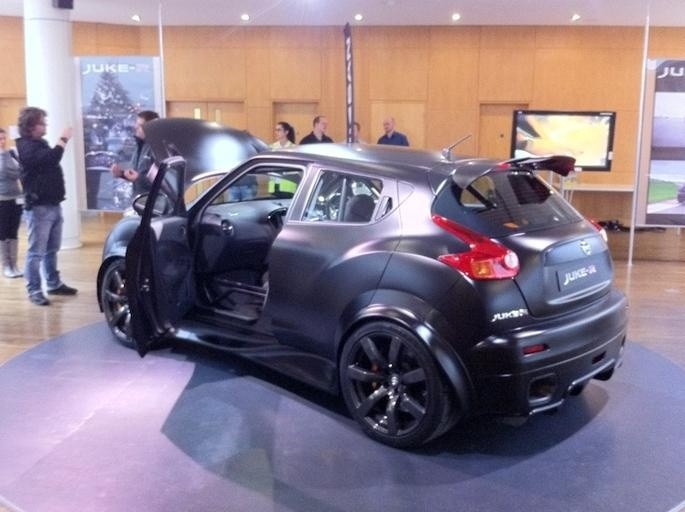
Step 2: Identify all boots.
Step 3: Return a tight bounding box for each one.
[10,239,23,277]
[0,238,16,278]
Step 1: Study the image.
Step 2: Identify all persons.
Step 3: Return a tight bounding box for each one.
[342,122,367,145]
[268,122,299,196]
[377,117,409,146]
[300,115,334,144]
[0,129,25,278]
[111,111,160,201]
[14,106,77,306]
[227,175,258,202]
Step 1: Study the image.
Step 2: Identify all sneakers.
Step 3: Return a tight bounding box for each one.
[47,284,77,295]
[29,292,49,305]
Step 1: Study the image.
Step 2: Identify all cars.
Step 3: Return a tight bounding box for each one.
[97,118,629,444]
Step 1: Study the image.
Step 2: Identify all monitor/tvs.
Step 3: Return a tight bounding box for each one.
[510,109,615,171]
